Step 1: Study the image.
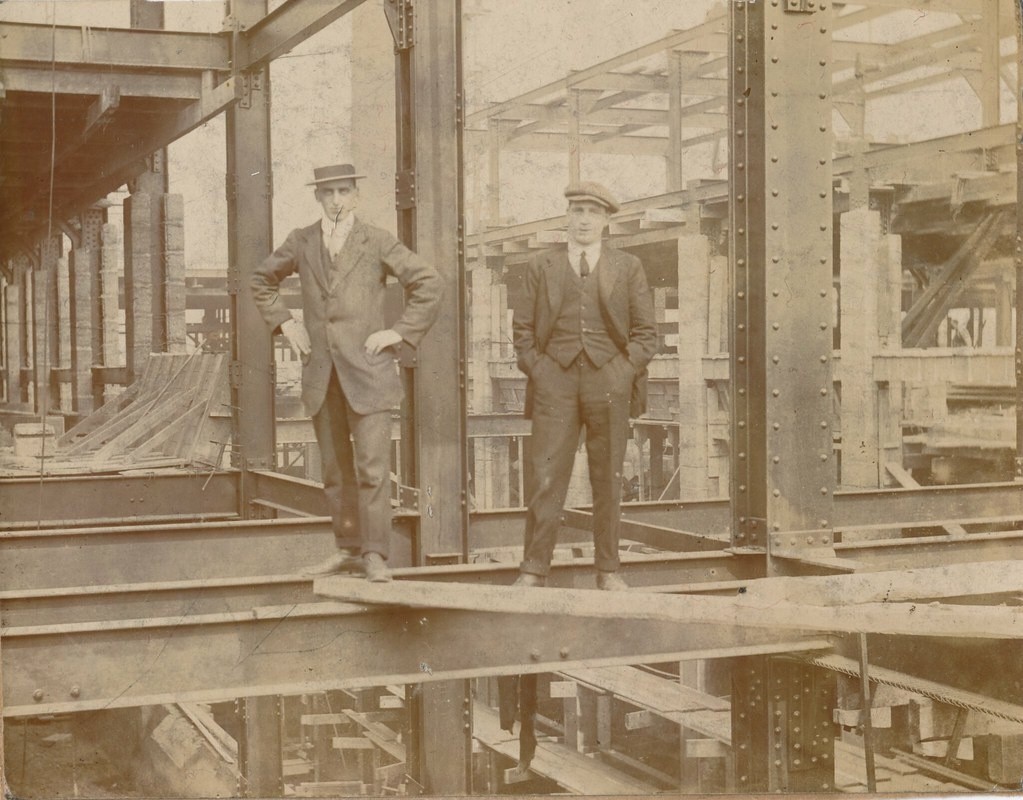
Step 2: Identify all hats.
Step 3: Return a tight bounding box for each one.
[565,182,621,214]
[306,163,367,186]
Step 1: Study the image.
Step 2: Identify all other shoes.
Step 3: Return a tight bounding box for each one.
[511,573,548,587]
[299,549,367,578]
[362,551,393,582]
[597,569,628,592]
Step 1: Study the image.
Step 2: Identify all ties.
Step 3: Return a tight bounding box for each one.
[580,252,589,279]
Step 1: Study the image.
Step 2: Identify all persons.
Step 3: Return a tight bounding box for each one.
[512,180,659,588]
[249,163,447,583]
